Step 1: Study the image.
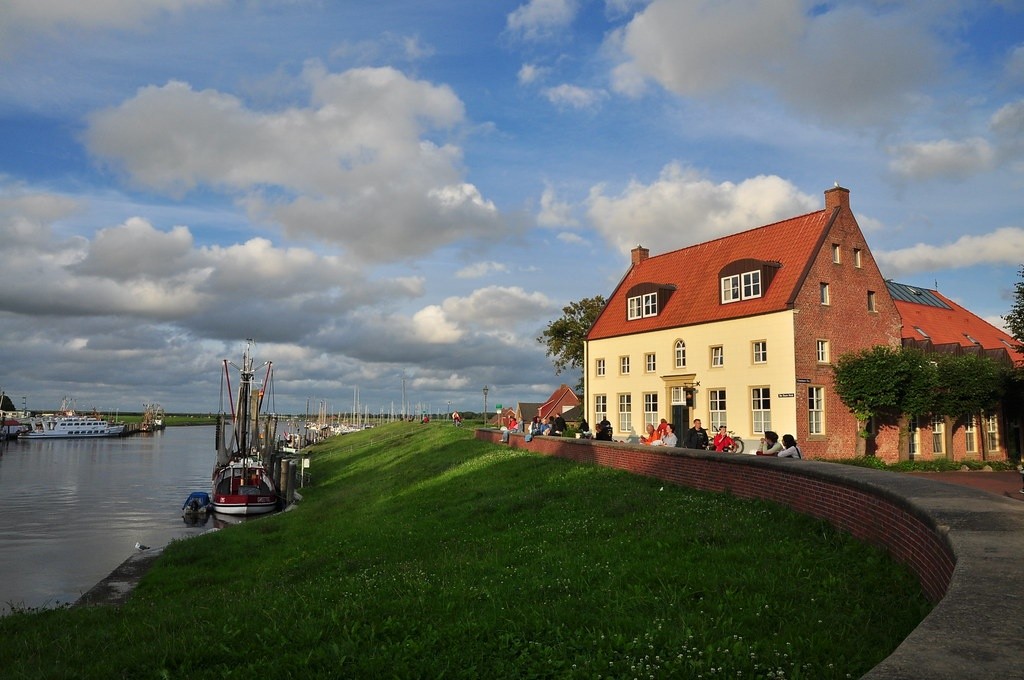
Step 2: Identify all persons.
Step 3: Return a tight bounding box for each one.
[421,416,429,424]
[683,419,709,450]
[758,430,782,455]
[657,419,668,436]
[518,416,524,431]
[595,416,613,442]
[527,412,567,436]
[660,424,677,447]
[190,498,199,512]
[713,426,737,453]
[451,411,461,422]
[640,424,660,445]
[777,434,802,459]
[499,417,517,442]
[579,419,589,432]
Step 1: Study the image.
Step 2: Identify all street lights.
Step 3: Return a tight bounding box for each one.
[483,384,488,428]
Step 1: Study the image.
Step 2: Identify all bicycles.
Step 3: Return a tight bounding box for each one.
[705,425,744,455]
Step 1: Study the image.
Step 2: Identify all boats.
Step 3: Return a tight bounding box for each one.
[13,396,126,439]
[212,340,281,516]
[0,417,29,442]
[140,403,167,433]
[182,491,213,515]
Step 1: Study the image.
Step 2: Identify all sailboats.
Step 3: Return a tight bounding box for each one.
[279,396,423,454]
